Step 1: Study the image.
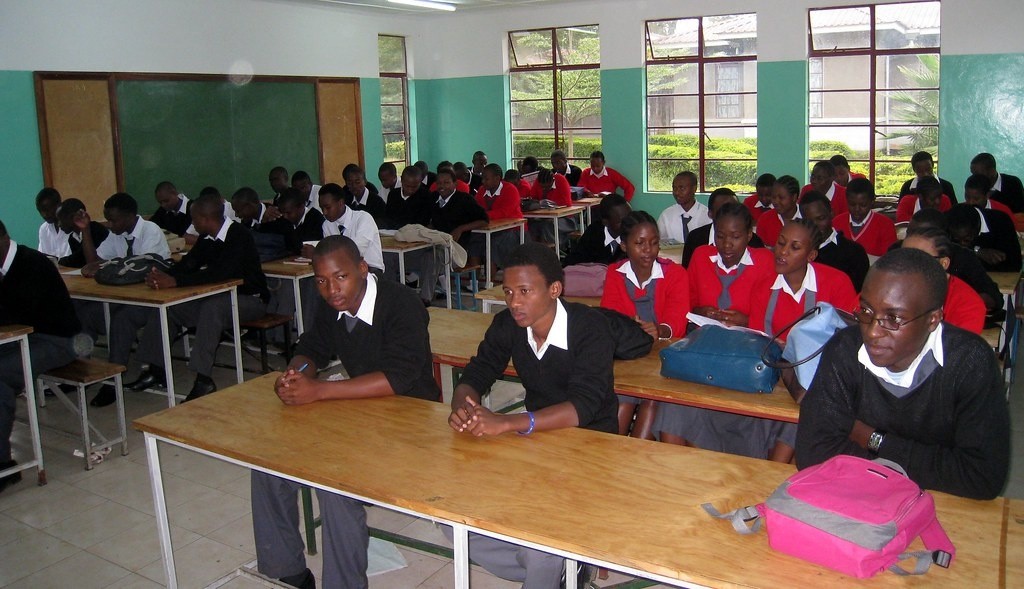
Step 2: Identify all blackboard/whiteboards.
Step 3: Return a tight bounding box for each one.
[108,72,325,222]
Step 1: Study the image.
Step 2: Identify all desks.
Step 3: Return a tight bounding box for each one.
[130,371,1024,589]
[426,305,800,424]
[58,265,244,407]
[0,324,47,486]
[659,242,1024,311]
[170,244,315,336]
[474,277,1003,353]
[470,218,528,288]
[574,197,603,225]
[523,205,587,262]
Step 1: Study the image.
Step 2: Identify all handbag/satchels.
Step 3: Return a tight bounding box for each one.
[658,324,783,394]
[559,262,608,299]
[761,301,861,405]
[161,228,186,253]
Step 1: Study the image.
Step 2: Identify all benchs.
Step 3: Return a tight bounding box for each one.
[171,313,292,374]
[407,264,480,311]
[37,358,130,471]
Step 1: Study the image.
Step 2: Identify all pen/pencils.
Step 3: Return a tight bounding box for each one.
[299,363,309,372]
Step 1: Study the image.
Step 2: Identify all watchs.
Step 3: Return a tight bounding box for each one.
[973,246,980,255]
[867,430,886,455]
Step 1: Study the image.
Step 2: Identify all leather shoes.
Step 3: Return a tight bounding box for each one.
[180,380,217,404]
[121,371,175,393]
[44,383,90,396]
[90,384,118,406]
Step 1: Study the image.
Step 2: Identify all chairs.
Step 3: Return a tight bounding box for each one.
[381,236,453,309]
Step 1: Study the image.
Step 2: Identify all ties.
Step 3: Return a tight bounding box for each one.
[681,214,692,245]
[715,263,744,310]
[125,236,135,258]
[763,288,816,346]
[624,275,657,326]
[339,224,345,236]
[611,240,619,252]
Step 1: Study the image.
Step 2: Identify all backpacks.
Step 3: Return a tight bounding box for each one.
[570,186,585,200]
[520,196,540,211]
[94,253,179,285]
[393,222,467,273]
[252,233,289,263]
[701,450,957,579]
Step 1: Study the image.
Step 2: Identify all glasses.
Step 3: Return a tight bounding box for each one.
[852,303,939,331]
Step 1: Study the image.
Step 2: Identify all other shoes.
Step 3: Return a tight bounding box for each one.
[461,285,477,293]
[277,342,298,357]
[436,290,448,300]
[406,279,421,291]
[271,568,316,589]
[0,459,22,492]
[560,558,583,589]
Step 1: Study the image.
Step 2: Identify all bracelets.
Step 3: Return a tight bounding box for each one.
[518,410,534,435]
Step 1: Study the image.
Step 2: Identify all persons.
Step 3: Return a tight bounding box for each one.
[0,149,1024,589]
